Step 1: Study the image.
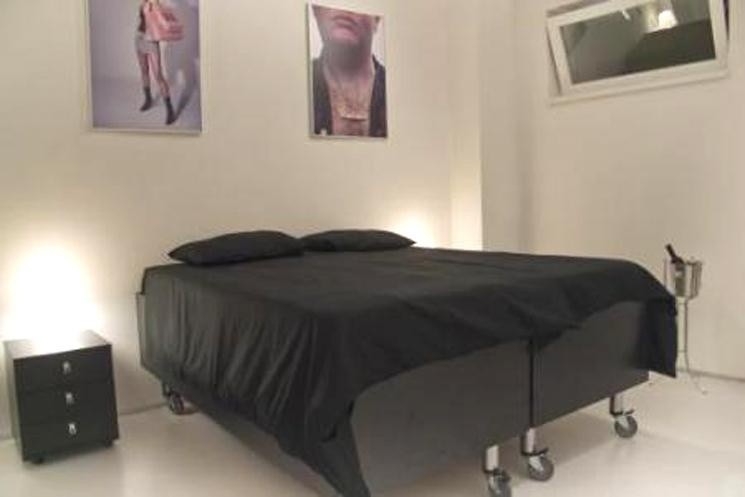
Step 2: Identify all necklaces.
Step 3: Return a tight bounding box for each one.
[324,52,376,120]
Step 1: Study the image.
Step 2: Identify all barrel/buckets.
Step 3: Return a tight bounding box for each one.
[661,258,702,298]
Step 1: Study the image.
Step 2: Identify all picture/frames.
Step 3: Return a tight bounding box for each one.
[85,1,207,136]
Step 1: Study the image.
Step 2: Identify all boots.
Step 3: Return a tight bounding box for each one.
[139,87,154,110]
[162,97,177,124]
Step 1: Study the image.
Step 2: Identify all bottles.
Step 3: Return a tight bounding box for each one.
[662,243,686,264]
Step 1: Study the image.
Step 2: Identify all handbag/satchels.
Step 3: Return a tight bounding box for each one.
[146,6,183,41]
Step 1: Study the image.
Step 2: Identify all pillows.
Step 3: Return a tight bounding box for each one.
[168,230,304,267]
[295,227,416,252]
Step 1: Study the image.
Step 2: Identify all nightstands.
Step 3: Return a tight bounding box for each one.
[3,329,119,466]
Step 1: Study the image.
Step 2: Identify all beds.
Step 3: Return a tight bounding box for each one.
[135,244,679,497]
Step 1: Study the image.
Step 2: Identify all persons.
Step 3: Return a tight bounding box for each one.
[133,0,178,126]
